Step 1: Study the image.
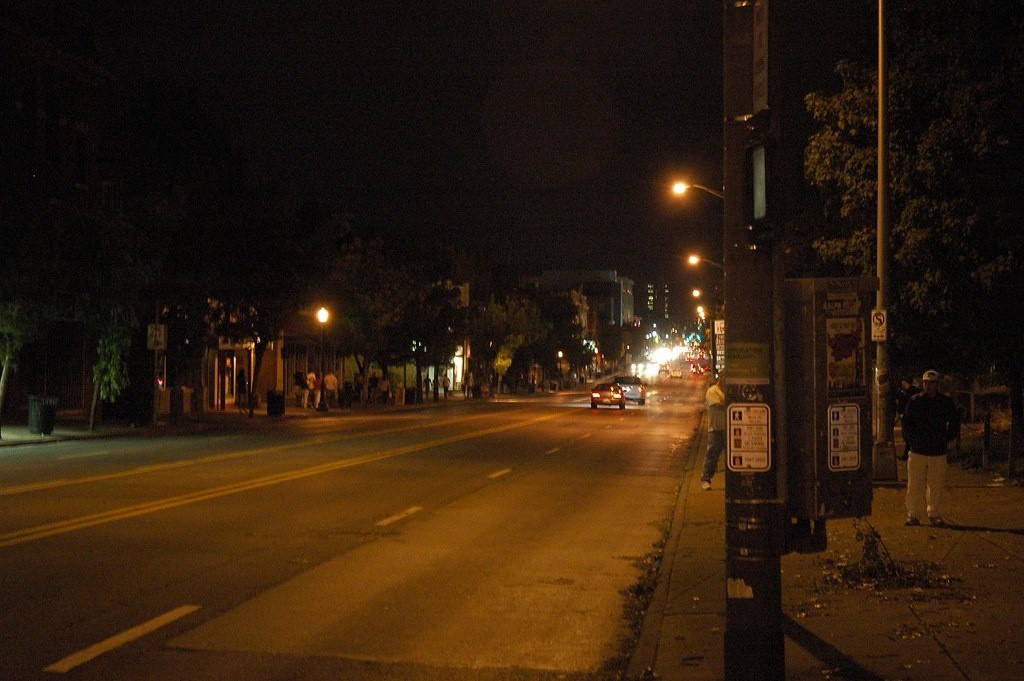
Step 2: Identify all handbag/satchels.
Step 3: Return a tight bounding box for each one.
[388,391,393,399]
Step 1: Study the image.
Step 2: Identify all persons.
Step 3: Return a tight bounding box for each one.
[895,369,961,527]
[700,371,728,490]
[237,367,247,416]
[292,366,544,410]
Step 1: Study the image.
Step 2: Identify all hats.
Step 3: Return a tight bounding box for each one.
[902,375,913,384]
[923,370,940,382]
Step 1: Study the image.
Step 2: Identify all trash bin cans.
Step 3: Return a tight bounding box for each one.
[405,387,416,404]
[27,393,59,436]
[266,389,286,417]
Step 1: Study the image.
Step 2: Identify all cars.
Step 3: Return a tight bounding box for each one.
[669,368,682,379]
[590,383,627,410]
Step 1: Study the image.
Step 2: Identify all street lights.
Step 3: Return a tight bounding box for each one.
[316,305,331,412]
[594,346,598,377]
[558,350,564,392]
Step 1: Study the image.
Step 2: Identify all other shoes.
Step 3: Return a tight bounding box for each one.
[904,518,920,526]
[899,455,908,461]
[702,481,711,490]
[928,518,946,528]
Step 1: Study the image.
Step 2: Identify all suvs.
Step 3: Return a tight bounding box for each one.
[613,375,649,405]
